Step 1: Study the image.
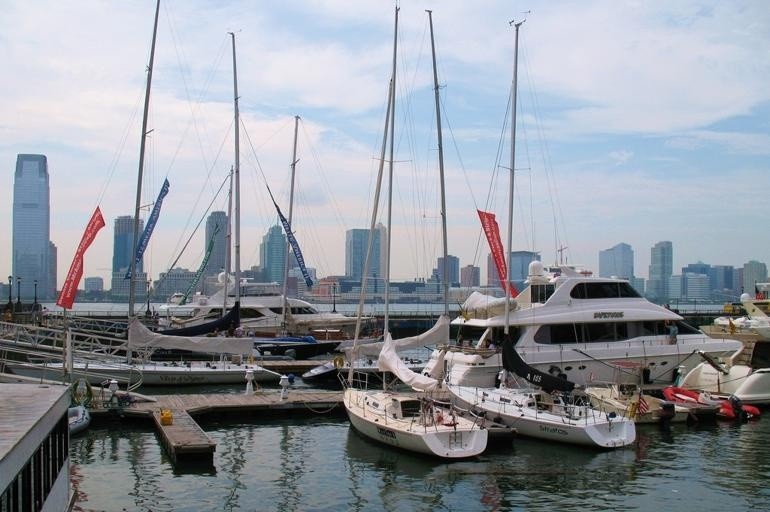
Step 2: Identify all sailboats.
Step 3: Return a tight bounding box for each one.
[448,20,637,448]
[341,3,488,459]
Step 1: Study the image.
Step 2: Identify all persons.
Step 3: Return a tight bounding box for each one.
[42,306,49,324]
[664,320,679,344]
[457,334,497,349]
[5,309,12,327]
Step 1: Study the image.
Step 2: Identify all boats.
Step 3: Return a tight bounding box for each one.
[588,367,770,423]
[697,292,770,345]
[67,404,92,434]
[724,280,769,315]
[302,261,745,396]
